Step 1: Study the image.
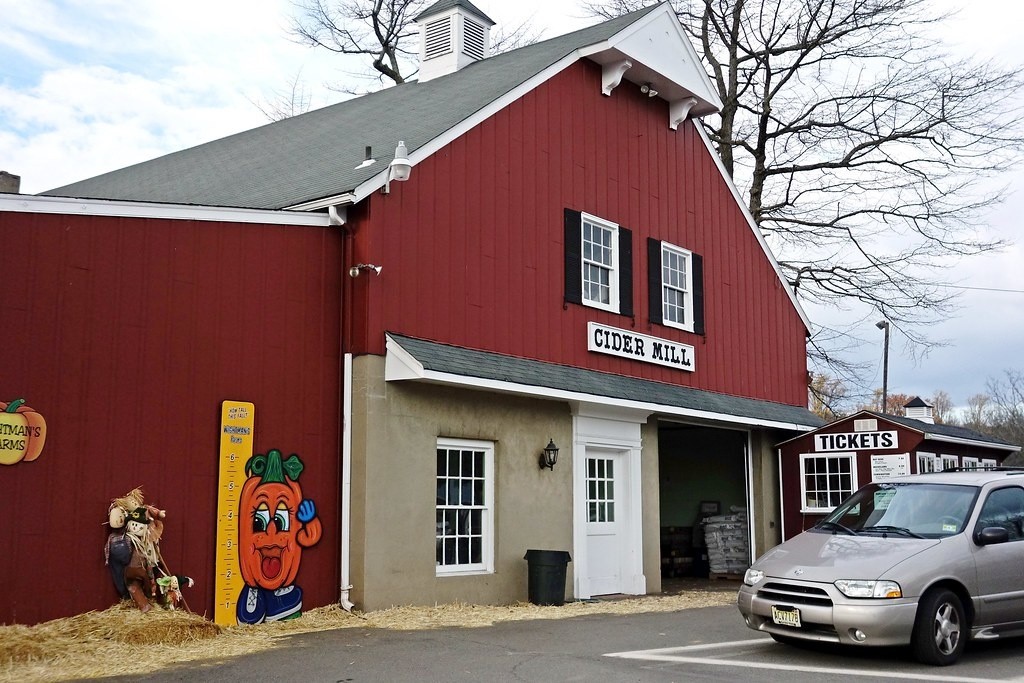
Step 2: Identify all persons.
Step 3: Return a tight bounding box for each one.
[104,488,193,612]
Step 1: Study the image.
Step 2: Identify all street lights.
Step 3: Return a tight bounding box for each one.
[876,320,891,414]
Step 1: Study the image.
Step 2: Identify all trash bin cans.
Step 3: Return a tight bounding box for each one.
[522,549,572,606]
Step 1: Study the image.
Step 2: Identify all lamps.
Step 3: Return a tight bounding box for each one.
[538,437,559,472]
[380,138,411,194]
[641,83,658,98]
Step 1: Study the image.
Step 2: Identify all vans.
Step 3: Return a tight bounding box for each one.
[737,469,1024,666]
